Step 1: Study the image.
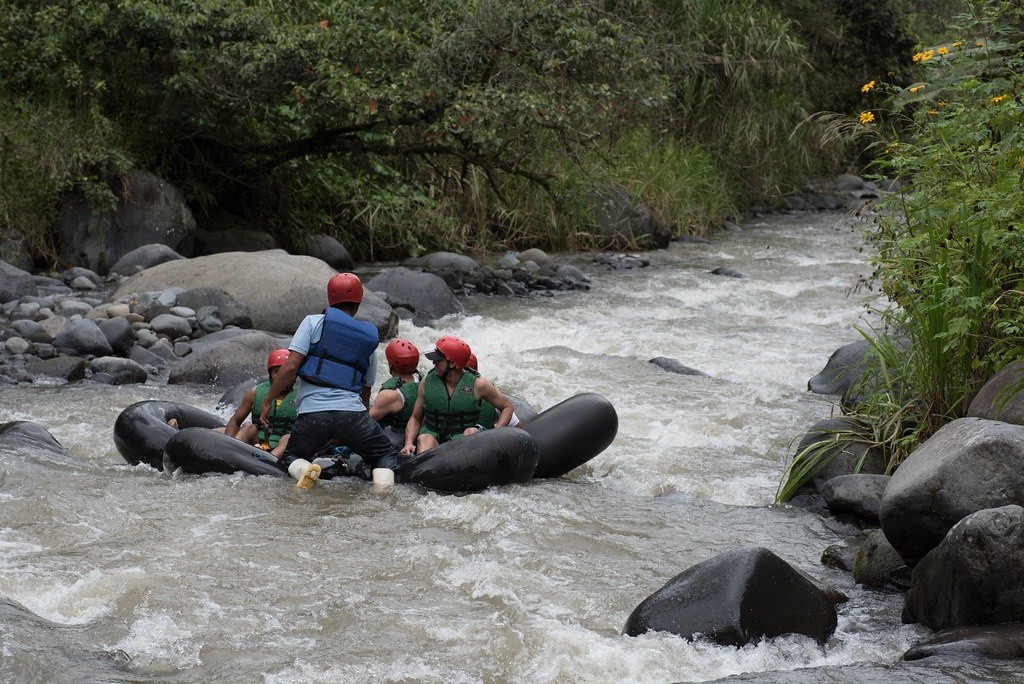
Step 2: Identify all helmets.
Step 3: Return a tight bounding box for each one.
[436,336,471,367]
[385,339,419,373]
[466,354,477,370]
[328,273,363,306]
[267,349,291,374]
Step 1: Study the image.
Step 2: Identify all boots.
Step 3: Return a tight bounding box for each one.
[288,458,321,490]
[372,468,394,484]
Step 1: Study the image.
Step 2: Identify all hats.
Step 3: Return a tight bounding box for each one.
[425,349,446,361]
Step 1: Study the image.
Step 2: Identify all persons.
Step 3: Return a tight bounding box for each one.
[211,336,526,456]
[260,272,399,492]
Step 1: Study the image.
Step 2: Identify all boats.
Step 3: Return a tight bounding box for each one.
[115,371,618,496]
